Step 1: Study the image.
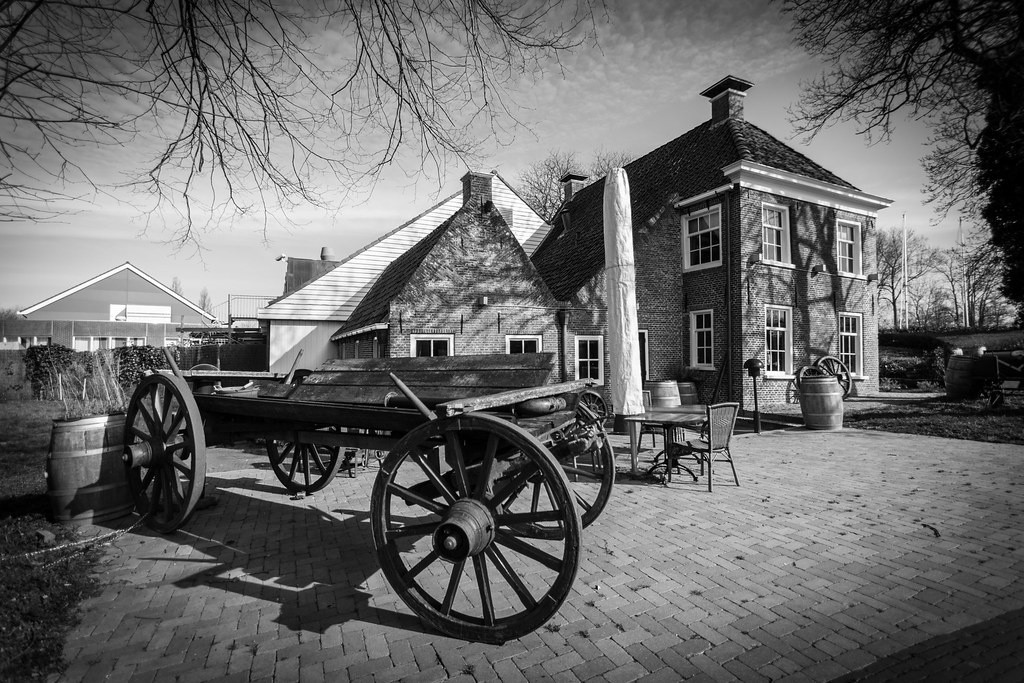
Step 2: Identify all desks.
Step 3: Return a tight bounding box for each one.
[646,404,707,463]
[624,412,708,483]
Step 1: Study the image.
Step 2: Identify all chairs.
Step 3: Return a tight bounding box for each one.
[668,402,739,491]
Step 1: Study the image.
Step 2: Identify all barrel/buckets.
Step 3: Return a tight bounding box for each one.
[43,410,132,522]
[214,384,260,398]
[945,354,980,399]
[799,375,843,429]
[643,381,700,407]
